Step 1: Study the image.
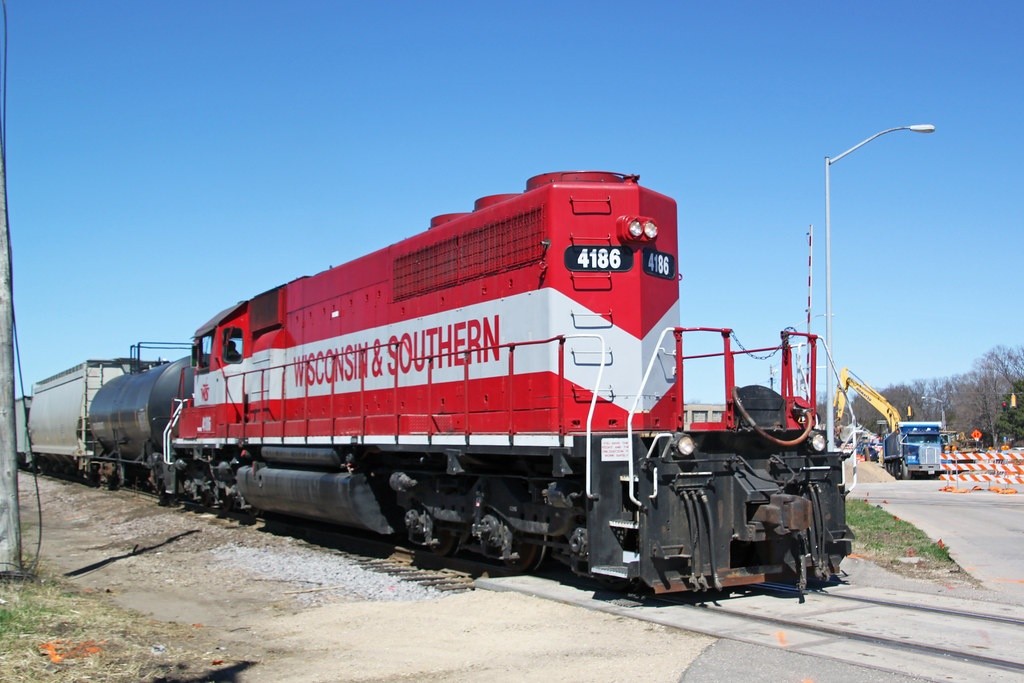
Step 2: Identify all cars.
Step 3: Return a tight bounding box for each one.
[874,443,883,452]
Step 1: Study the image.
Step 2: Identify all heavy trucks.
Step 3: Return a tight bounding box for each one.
[882,421,941,480]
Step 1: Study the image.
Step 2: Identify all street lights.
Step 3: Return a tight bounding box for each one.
[824,125,935,451]
[920,397,946,430]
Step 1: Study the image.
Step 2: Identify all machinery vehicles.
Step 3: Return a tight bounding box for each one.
[833,367,957,462]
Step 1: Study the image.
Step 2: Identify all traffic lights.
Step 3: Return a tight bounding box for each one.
[1002,401,1006,412]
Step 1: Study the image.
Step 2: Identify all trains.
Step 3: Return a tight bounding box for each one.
[15,171,855,604]
